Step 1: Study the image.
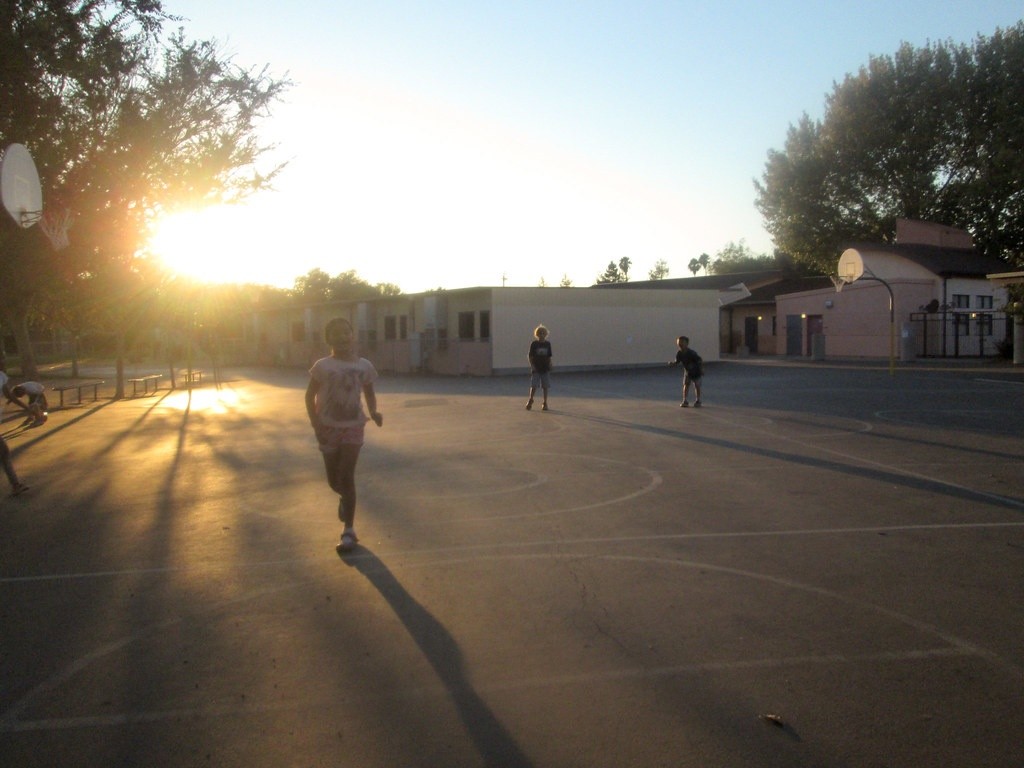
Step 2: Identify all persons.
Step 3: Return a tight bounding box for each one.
[6,382,47,428]
[667,336,704,407]
[304,318,384,553]
[524,326,552,413]
[0,371,35,499]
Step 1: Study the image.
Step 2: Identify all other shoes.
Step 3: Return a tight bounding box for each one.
[541,402,548,410]
[680,400,689,406]
[694,401,702,407]
[12,481,29,497]
[524,399,535,410]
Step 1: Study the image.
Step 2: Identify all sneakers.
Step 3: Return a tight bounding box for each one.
[334,534,359,551]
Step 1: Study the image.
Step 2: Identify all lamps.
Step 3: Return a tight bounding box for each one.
[801,312,806,319]
[757,315,762,321]
[1014,302,1022,307]
[971,313,976,318]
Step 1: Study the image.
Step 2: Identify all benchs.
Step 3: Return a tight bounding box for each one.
[182,371,202,382]
[52,379,105,406]
[127,374,162,395]
